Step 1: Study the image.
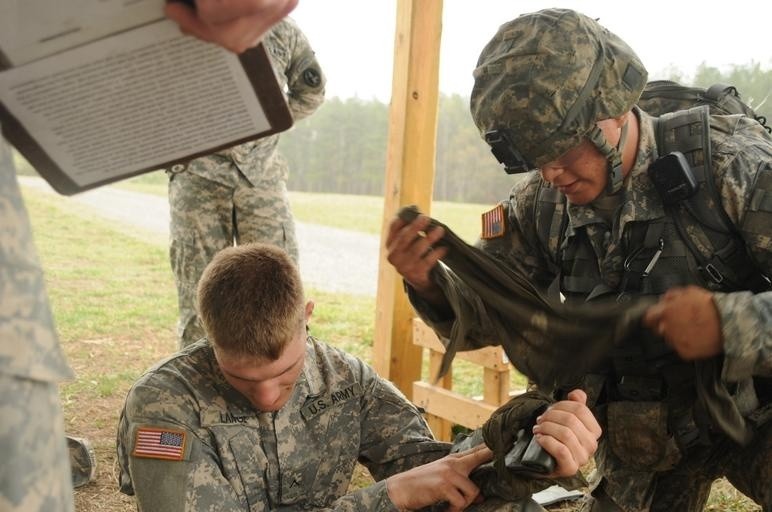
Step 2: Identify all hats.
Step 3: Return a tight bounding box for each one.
[66,435,97,490]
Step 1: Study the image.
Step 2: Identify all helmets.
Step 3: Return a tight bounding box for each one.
[469,8,650,177]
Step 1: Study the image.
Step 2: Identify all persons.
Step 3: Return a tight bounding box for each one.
[171,18,325,350]
[2,0,301,511]
[117,240,604,511]
[386,9,772,510]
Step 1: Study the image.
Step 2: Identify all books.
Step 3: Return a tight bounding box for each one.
[2,1,292,201]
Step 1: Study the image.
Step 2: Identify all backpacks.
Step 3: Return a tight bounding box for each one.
[636,80,772,292]
